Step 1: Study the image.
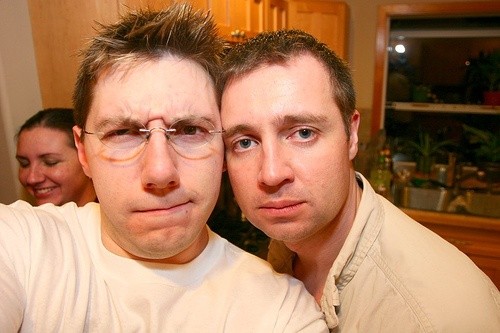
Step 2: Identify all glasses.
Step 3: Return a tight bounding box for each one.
[82,117,226,150]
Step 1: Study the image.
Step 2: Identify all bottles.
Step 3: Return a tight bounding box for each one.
[384,150,397,197]
[370,151,392,202]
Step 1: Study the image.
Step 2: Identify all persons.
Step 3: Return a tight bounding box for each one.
[217,27,499,332]
[12,107,100,208]
[0,1,329,333]
